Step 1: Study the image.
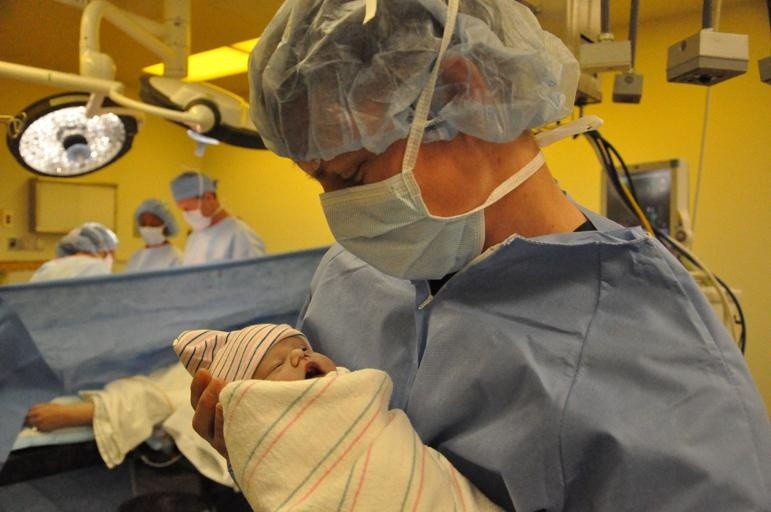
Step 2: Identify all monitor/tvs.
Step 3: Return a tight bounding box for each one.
[602,158,691,251]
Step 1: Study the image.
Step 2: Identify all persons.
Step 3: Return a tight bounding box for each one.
[123,169,267,275]
[29,222,119,282]
[25,397,101,433]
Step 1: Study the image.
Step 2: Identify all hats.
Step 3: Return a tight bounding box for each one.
[58,223,118,251]
[171,172,216,202]
[173,323,312,386]
[134,199,178,236]
[248,0,581,161]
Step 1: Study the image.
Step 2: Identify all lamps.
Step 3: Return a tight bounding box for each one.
[0,0,269,179]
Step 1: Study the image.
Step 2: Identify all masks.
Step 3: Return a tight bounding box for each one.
[318,123,497,281]
[139,226,167,245]
[182,208,211,231]
[104,254,113,270]
